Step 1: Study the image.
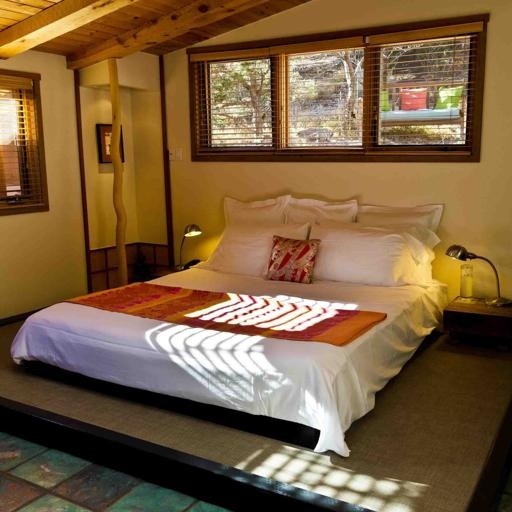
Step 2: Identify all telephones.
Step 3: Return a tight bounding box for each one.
[184,259,200,270]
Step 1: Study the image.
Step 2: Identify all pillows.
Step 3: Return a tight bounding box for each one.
[188,224,308,278]
[222,194,290,225]
[307,221,440,288]
[357,203,445,234]
[265,235,321,281]
[283,196,358,227]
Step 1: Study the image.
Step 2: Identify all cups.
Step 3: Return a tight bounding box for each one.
[460,264,473,298]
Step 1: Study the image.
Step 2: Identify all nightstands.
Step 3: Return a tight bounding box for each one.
[443,295,512,357]
[138,262,174,279]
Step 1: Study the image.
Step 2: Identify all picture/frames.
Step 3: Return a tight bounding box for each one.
[97,122,126,164]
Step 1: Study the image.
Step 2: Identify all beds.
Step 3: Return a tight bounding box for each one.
[10,192,450,457]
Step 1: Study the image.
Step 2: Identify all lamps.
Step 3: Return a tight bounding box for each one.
[445,245,512,307]
[173,223,204,271]
